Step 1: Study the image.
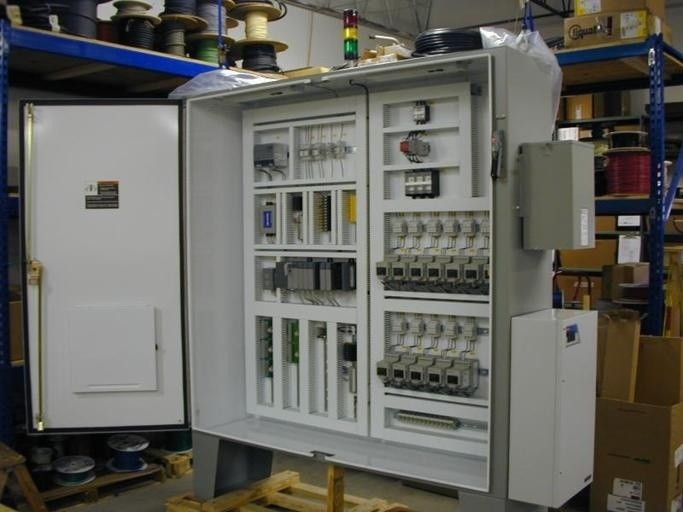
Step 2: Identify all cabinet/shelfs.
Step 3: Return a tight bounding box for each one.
[1,19,284,450]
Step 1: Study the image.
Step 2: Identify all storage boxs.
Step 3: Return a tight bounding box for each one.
[9,300,25,362]
[556,0,683,512]
